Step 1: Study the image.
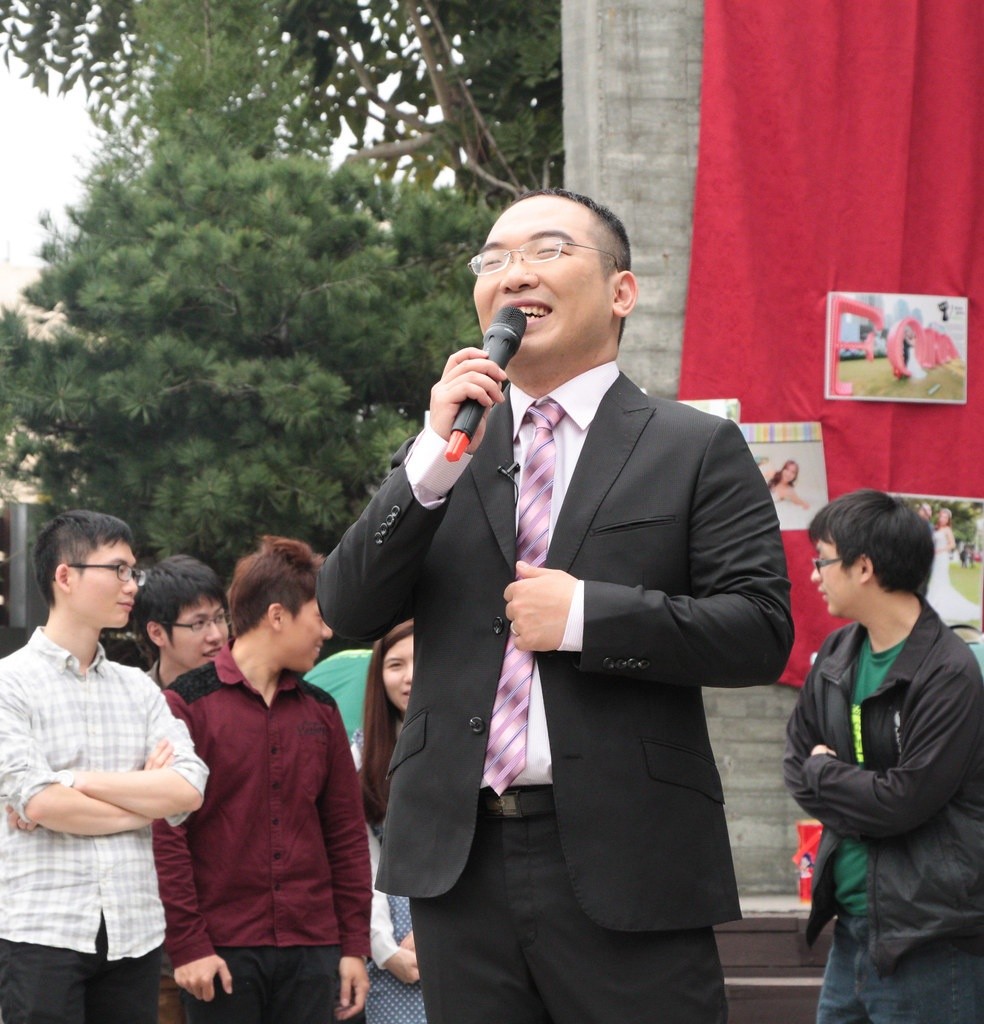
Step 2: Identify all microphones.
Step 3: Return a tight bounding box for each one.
[446,306,526,463]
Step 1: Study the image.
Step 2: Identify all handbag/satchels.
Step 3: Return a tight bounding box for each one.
[790,819,825,907]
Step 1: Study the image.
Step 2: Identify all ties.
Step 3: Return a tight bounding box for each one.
[482,402,566,797]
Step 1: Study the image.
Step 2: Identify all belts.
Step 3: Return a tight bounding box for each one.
[478,791,554,819]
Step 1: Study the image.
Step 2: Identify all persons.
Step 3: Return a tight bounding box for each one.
[901,331,927,378]
[761,461,808,511]
[781,490,984,1024]
[316,189,795,1024]
[915,502,982,628]
[0,509,431,1024]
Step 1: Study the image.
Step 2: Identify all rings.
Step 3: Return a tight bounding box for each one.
[509,620,522,638]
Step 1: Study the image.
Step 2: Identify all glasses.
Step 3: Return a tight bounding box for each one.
[467,236,620,278]
[812,556,843,574]
[159,606,233,633]
[52,563,147,587]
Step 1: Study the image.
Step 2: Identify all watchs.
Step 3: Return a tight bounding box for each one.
[56,770,76,788]
